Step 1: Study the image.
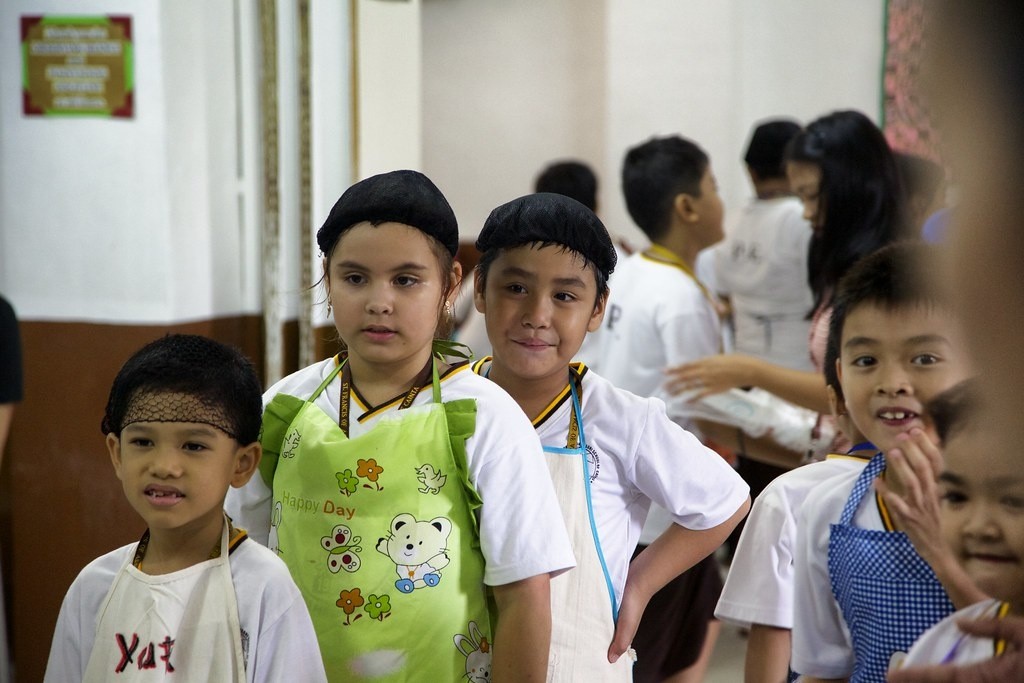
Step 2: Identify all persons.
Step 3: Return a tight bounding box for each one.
[43,332,327,683]
[468,194,752,683]
[446,111,1024,683]
[223,169,576,683]
[1,295,25,683]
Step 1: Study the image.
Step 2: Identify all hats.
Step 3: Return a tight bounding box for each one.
[316,170,461,257]
[476,191,617,282]
